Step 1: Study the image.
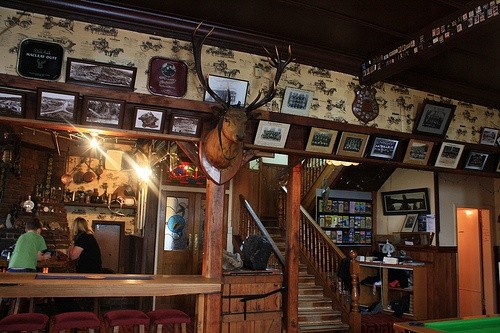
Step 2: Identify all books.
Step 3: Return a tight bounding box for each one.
[319,200,372,243]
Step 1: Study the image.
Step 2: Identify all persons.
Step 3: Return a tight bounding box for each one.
[288,93,306,109]
[3,218,51,303]
[374,140,395,155]
[261,130,281,140]
[312,135,330,146]
[53,217,102,313]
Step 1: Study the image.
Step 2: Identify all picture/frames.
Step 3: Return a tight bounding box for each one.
[36,88,79,123]
[129,106,166,133]
[167,114,203,137]
[305,128,338,156]
[480,127,500,146]
[280,86,314,116]
[381,188,431,215]
[413,100,457,139]
[252,120,291,149]
[0,92,26,118]
[78,94,126,129]
[66,57,137,92]
[400,214,419,232]
[337,132,488,171]
[204,74,249,108]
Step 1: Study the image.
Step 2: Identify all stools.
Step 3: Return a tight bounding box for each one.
[0,309,189,333]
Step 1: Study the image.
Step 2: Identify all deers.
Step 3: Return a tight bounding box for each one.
[190,20,297,168]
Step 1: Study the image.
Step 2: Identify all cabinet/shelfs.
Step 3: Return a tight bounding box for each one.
[357,258,434,322]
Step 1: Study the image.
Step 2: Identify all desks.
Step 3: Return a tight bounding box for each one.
[0,251,68,273]
[393,316,500,333]
